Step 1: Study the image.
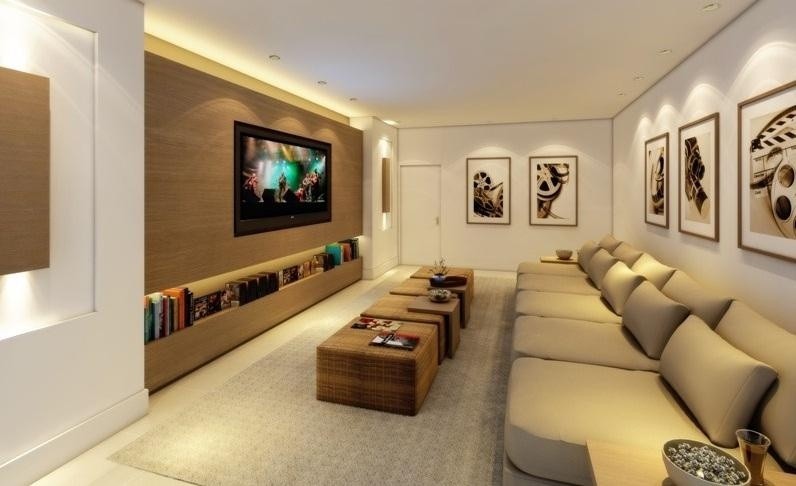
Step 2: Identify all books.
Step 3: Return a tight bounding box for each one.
[351,317,404,333]
[368,329,419,351]
[144,238,360,347]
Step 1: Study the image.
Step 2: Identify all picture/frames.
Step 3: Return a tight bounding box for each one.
[466,156,512,224]
[678,109,720,244]
[528,155,579,227]
[644,132,669,229]
[736,80,796,265]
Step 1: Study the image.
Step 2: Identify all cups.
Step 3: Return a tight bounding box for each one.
[735,427,771,486]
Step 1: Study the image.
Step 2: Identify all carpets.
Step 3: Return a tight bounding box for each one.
[103,265,515,486]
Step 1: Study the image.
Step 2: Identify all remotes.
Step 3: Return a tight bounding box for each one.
[381,333,394,343]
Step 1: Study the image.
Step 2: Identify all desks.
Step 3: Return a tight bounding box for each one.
[540,256,578,263]
[583,437,789,485]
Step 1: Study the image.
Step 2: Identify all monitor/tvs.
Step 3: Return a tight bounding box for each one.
[234,120,332,237]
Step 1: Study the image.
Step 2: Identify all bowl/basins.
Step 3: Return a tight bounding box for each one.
[661,439,751,486]
[556,250,573,260]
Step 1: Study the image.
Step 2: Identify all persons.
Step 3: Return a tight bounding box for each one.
[279,171,287,201]
[296,168,320,199]
[249,173,258,190]
